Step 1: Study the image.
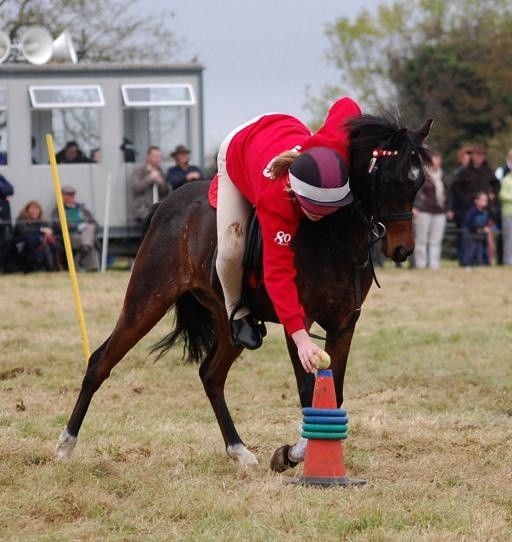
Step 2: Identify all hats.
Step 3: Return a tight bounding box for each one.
[285,143,355,209]
[463,145,488,155]
[170,143,193,158]
[62,185,76,194]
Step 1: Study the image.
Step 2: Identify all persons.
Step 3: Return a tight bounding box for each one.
[11,199,64,271]
[168,144,206,190]
[410,144,511,268]
[0,173,14,273]
[131,145,169,225]
[215,96,362,374]
[90,147,101,161]
[51,184,102,271]
[49,139,97,163]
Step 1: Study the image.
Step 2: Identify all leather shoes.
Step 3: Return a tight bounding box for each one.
[227,309,265,353]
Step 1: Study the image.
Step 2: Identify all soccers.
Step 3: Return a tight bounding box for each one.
[313,352,331,369]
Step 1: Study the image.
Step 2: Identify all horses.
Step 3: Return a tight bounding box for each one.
[51,105,434,474]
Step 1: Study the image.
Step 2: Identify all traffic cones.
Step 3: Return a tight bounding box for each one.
[285,367,365,485]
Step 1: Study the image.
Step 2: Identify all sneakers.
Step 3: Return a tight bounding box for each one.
[78,244,92,260]
[382,259,410,270]
